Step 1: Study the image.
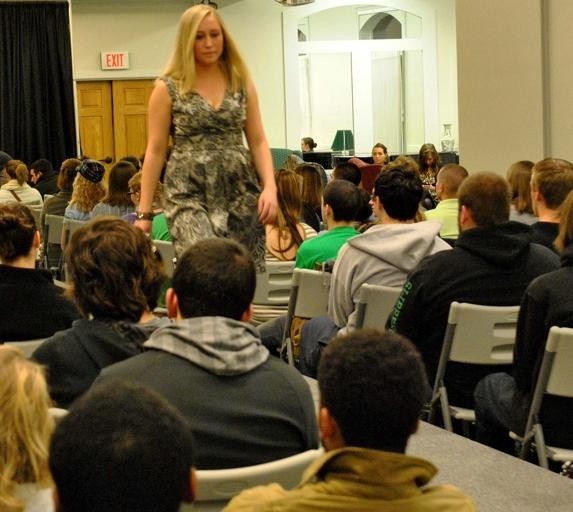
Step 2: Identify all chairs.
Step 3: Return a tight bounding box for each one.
[0,184,573,511]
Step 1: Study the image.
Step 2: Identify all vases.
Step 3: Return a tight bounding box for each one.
[440,123,454,151]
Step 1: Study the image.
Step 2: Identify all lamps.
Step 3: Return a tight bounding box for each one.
[330,128,353,157]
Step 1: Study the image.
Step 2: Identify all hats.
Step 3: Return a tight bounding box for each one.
[74,158,104,183]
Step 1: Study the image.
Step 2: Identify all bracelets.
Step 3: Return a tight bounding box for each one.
[136,210,154,222]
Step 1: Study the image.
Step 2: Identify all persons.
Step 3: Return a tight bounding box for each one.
[0,342,56,511]
[417,144,444,207]
[223,329,477,510]
[29,214,175,409]
[1,199,76,343]
[47,379,197,511]
[301,139,318,151]
[257,152,572,476]
[130,3,280,275]
[372,143,390,165]
[1,150,167,283]
[87,236,319,470]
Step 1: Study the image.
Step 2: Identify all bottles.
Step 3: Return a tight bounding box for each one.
[440,123,455,152]
